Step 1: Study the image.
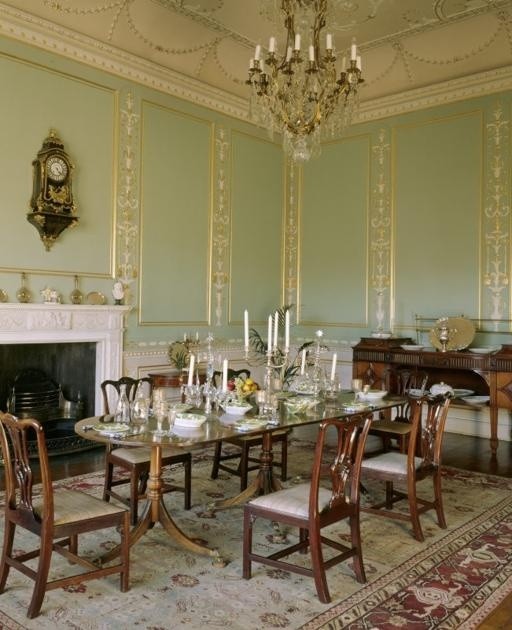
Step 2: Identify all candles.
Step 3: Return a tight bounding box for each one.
[301,349,307,375]
[274,309,279,348]
[243,309,251,351]
[267,314,272,355]
[186,355,195,386]
[285,309,290,349]
[223,359,228,390]
[329,352,337,382]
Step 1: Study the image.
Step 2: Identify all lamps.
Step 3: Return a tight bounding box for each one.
[243,1,372,165]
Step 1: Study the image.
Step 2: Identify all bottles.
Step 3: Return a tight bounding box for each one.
[70,278,83,304]
[90,381,391,431]
[16,275,32,303]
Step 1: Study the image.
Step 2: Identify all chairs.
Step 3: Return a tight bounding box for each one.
[241,408,375,606]
[347,367,429,460]
[98,375,192,527]
[207,365,294,495]
[0,409,134,618]
[346,388,454,543]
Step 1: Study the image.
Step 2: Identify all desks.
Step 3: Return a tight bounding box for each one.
[69,386,421,569]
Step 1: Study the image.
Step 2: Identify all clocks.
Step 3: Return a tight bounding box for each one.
[21,128,81,253]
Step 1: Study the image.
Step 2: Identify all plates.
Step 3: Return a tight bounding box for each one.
[0,289,8,303]
[403,381,493,407]
[399,315,501,355]
[86,291,106,307]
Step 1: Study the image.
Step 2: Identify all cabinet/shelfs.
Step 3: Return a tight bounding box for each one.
[348,335,511,466]
[150,367,221,408]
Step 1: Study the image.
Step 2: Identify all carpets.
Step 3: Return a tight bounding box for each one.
[0,437,512,630]
